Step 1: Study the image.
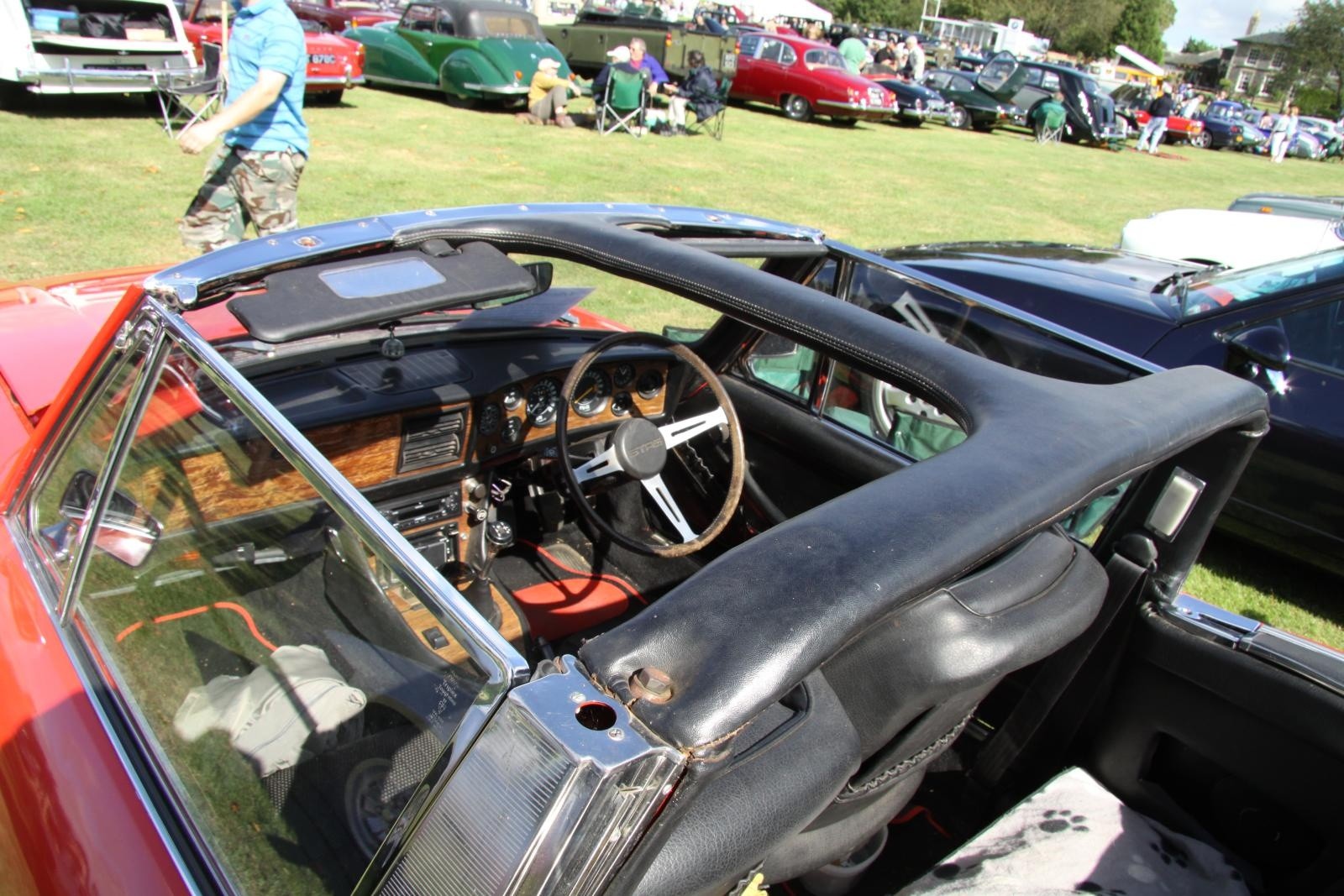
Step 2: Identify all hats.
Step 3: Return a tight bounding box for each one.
[1164,86,1173,93]
[538,58,561,70]
[606,45,630,63]
[1051,92,1064,102]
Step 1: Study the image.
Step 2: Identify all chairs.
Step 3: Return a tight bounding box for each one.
[680,73,733,141]
[1031,106,1067,145]
[591,65,650,140]
[308,501,1117,896]
[1320,136,1344,164]
[155,40,224,139]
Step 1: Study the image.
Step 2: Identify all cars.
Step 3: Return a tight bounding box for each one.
[1,0,1343,164]
[0,194,1344,896]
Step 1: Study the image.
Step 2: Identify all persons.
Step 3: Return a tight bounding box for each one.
[663,50,719,134]
[838,27,870,72]
[178,0,310,255]
[528,57,579,127]
[667,5,678,23]
[1136,86,1172,154]
[875,37,898,72]
[905,35,925,79]
[956,38,982,58]
[592,45,644,131]
[629,37,669,97]
[1033,92,1066,133]
[1174,80,1204,145]
[1259,106,1298,162]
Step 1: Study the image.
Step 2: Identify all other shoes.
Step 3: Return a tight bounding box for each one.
[556,116,575,127]
[660,122,685,136]
